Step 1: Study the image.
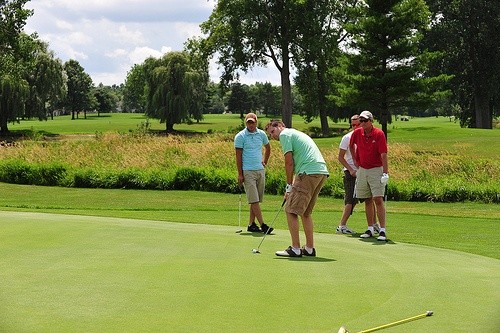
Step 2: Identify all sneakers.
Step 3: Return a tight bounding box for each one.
[261,223,273,234]
[248,222,263,232]
[337,226,355,234]
[373,224,380,233]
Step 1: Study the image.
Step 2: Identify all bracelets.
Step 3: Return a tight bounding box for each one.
[262,162,266,167]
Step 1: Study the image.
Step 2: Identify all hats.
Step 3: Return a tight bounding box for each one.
[358,111,372,121]
[246,113,257,123]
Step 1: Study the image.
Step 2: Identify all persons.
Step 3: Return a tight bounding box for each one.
[234,113,274,235]
[349,111,389,241]
[265,119,330,257]
[336,115,381,235]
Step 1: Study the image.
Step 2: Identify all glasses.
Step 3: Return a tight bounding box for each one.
[360,119,369,123]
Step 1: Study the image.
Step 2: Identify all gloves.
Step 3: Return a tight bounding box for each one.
[380,173,389,184]
[286,183,291,192]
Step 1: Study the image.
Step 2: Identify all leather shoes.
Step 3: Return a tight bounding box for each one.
[276,246,302,257]
[360,230,374,238]
[301,247,315,256]
[377,231,386,240]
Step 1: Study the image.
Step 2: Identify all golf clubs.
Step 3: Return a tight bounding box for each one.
[252,199,286,253]
[385,185,388,242]
[235,186,242,233]
[338,310,433,333]
